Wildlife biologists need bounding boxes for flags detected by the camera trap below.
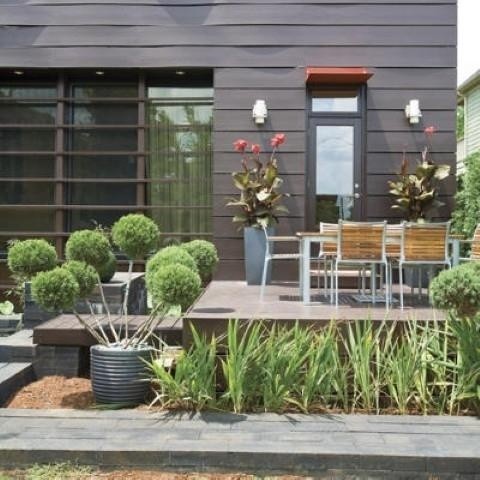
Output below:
[399,216,436,288]
[243,225,274,285]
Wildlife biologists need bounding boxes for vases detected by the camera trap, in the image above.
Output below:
[224,134,290,233]
[385,125,451,223]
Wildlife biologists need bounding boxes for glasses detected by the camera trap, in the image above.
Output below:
[404,99,422,124]
[253,98,268,124]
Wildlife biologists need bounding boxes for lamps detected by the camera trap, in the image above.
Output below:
[256,219,480,309]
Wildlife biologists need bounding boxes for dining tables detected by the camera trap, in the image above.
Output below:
[6,212,219,404]
